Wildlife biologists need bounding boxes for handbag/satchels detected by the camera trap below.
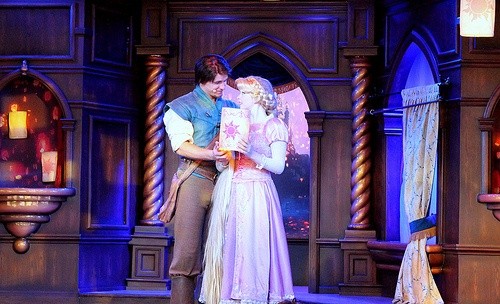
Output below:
[155,171,184,225]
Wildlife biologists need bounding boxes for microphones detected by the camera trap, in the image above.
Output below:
[204,85,217,93]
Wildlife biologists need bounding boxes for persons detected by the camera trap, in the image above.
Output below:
[199,76,296,304]
[158,55,240,303]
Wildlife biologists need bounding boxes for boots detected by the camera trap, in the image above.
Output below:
[168,274,194,303]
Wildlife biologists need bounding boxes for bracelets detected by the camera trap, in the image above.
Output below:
[255,153,267,170]
